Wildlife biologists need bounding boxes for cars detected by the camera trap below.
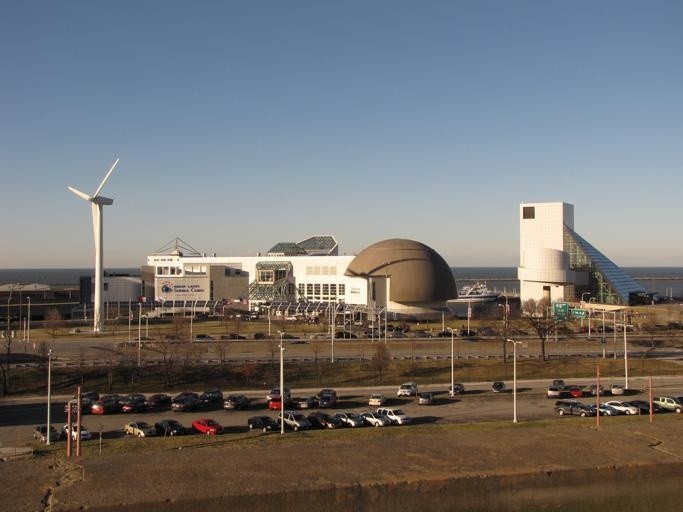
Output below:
[153,419,187,436]
[62,423,92,441]
[415,329,532,337]
[266,388,289,410]
[448,382,465,396]
[296,397,314,409]
[124,420,158,438]
[555,323,682,335]
[354,320,363,327]
[223,395,251,410]
[326,317,352,325]
[334,411,365,428]
[33,424,61,442]
[359,411,391,426]
[248,416,276,432]
[64,391,224,416]
[419,392,433,405]
[127,331,365,345]
[285,314,298,321]
[365,321,410,338]
[307,411,339,429]
[546,376,682,419]
[191,418,224,435]
[277,410,313,431]
[247,311,259,321]
[491,379,507,395]
[302,316,320,325]
[368,393,387,406]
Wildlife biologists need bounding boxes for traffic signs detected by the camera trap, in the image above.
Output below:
[552,302,590,321]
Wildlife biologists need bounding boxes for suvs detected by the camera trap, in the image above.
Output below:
[376,407,413,426]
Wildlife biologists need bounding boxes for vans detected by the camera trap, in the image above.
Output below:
[317,389,337,408]
[397,382,418,396]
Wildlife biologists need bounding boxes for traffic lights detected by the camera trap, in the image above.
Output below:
[24,295,33,344]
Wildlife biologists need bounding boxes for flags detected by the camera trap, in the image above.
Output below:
[0,330,8,339]
[158,296,165,304]
[139,296,149,303]
[9,330,17,339]
[222,298,232,305]
[240,297,248,305]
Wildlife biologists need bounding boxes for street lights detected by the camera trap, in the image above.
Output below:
[506,339,523,424]
[43,346,54,445]
[274,329,288,435]
[444,327,458,397]
[616,322,635,390]
[136,302,142,367]
[579,291,596,340]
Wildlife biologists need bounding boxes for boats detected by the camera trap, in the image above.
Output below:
[457,281,501,298]
